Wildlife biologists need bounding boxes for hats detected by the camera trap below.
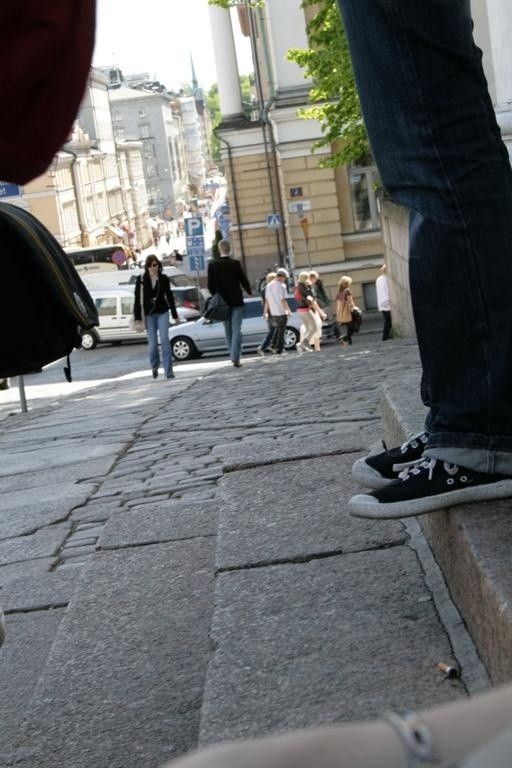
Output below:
[277,268,290,279]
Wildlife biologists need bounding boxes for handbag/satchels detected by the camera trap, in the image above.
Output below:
[336,299,352,324]
[129,314,146,333]
[202,292,230,320]
[0,201,100,377]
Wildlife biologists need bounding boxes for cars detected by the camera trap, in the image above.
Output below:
[197,187,217,214]
[148,292,341,361]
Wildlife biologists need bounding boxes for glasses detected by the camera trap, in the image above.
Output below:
[147,262,157,267]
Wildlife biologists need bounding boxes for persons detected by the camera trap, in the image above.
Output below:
[131,255,179,380]
[335,274,363,347]
[210,230,226,260]
[205,238,253,368]
[375,263,394,341]
[338,2,511,520]
[128,180,221,268]
[255,271,278,356]
[293,271,329,354]
[295,270,324,354]
[352,173,373,230]
[263,267,294,357]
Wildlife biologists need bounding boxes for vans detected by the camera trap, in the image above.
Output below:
[61,243,203,352]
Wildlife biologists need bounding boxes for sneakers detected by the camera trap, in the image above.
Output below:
[347,433,510,517]
[338,336,349,346]
[296,339,312,352]
[273,351,287,356]
[232,360,242,367]
[152,367,158,378]
[257,346,264,357]
[167,372,174,378]
